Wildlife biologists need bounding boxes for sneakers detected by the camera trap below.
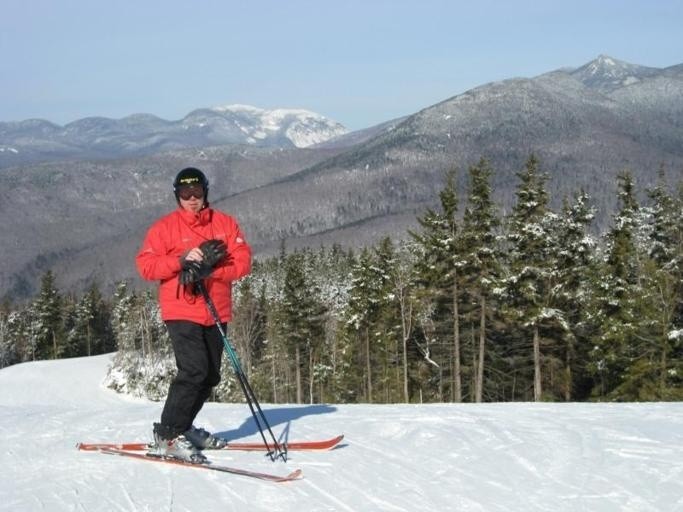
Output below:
[146,423,227,464]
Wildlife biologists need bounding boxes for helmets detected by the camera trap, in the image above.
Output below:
[173,168,208,192]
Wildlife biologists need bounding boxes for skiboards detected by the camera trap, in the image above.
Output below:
[77,431,345,481]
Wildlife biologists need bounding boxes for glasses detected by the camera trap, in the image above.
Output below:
[179,187,203,200]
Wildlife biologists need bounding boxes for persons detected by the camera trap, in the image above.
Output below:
[136,167,251,464]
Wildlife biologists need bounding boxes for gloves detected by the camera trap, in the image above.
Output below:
[179,239,226,286]
